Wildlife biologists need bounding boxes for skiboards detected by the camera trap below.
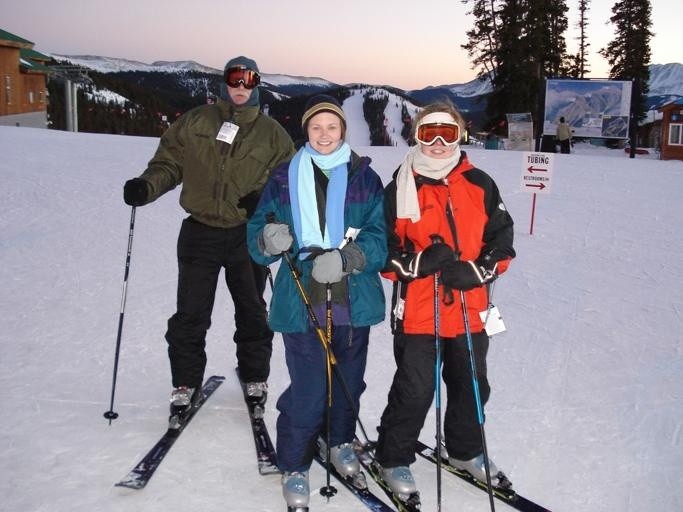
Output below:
[352,426,551,512]
[314,436,395,512]
[114,366,281,489]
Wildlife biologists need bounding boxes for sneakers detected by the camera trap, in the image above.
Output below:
[318,435,361,476]
[169,383,201,417]
[242,380,269,408]
[447,451,500,483]
[370,456,418,496]
[280,468,311,510]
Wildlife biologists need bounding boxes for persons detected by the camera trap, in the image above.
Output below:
[120,56,298,416]
[368,102,514,504]
[242,93,388,508]
[555,117,572,154]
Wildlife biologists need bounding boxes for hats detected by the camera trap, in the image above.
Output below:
[298,92,349,135]
[220,55,261,108]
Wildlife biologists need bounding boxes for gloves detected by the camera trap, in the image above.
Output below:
[439,251,500,292]
[122,176,150,208]
[235,188,264,221]
[390,241,457,286]
[257,221,296,258]
[310,240,368,286]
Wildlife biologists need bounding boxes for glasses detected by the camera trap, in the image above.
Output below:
[222,64,262,90]
[413,120,462,149]
